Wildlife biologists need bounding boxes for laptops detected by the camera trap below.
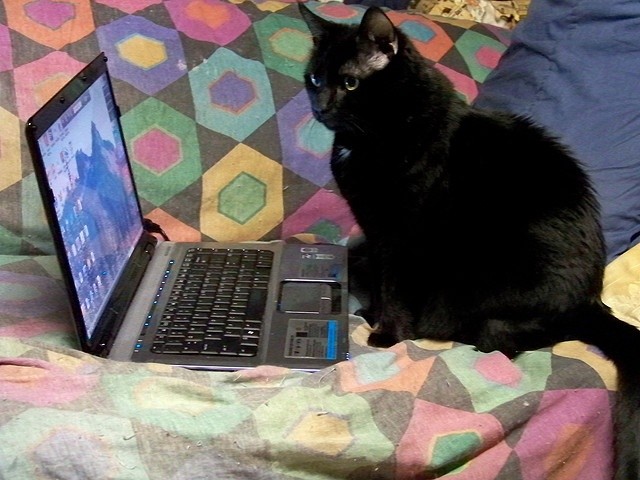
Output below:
[25,51,348,372]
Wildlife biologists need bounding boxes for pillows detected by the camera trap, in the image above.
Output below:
[469,1,640,263]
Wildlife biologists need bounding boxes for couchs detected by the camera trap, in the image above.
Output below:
[0,0,640,480]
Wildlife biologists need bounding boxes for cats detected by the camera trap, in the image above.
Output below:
[296,0,640,480]
